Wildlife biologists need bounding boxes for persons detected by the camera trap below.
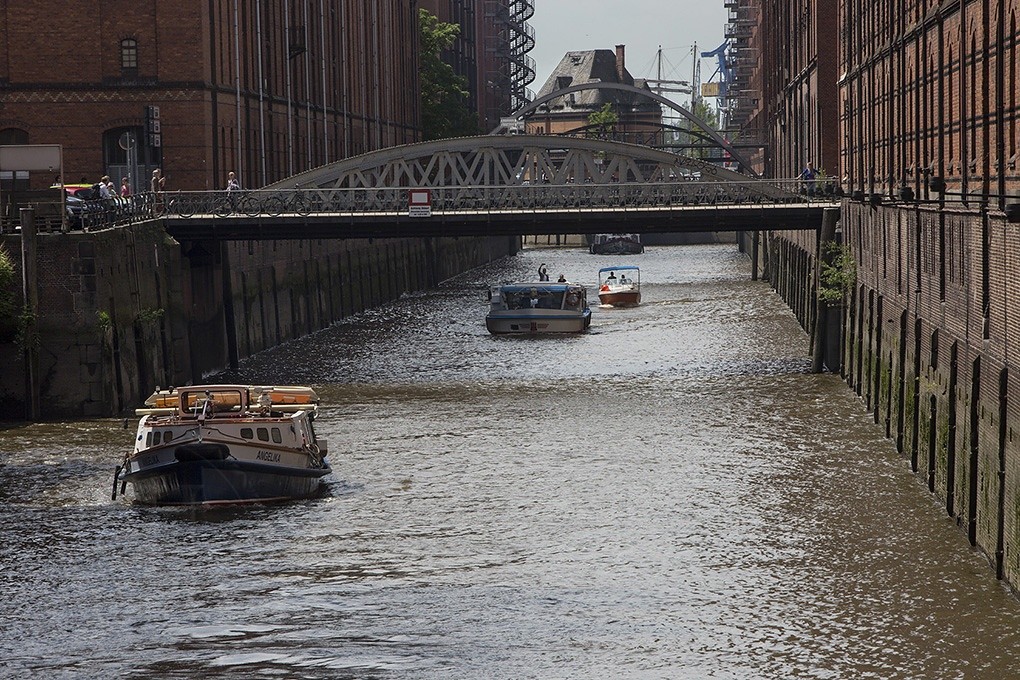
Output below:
[607,272,617,279]
[795,162,821,200]
[228,172,239,217]
[53,169,166,232]
[558,274,566,282]
[538,263,549,281]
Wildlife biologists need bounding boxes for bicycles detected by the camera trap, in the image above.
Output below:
[263,183,314,217]
[149,188,196,218]
[214,187,262,218]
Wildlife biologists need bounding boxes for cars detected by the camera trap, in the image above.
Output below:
[114,196,137,215]
[64,196,104,232]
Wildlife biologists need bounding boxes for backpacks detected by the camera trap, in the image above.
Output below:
[92,183,104,198]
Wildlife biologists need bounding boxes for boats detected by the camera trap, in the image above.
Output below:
[590,233,643,255]
[597,265,643,305]
[485,280,593,334]
[112,385,333,508]
